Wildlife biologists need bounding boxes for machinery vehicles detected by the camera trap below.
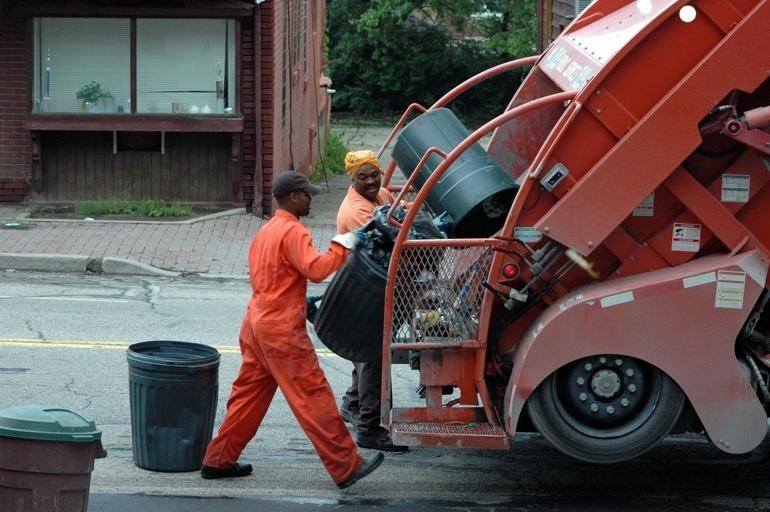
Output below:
[365,2,770,466]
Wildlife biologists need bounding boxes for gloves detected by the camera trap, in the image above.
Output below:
[306,295,322,323]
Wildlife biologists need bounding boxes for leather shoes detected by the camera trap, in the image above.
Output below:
[337,453,384,488]
[201,463,253,479]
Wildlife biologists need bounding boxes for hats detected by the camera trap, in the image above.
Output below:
[273,171,321,198]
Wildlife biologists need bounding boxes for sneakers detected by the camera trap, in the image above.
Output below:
[356,434,409,452]
[341,406,358,426]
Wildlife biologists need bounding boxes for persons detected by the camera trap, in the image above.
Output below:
[335,149,431,455]
[199,166,386,490]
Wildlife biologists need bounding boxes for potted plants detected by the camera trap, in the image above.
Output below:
[73,79,115,112]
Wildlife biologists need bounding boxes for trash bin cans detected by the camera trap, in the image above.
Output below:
[390,106,522,250]
[312,204,445,364]
[124,340,222,473]
[0,403,109,512]
